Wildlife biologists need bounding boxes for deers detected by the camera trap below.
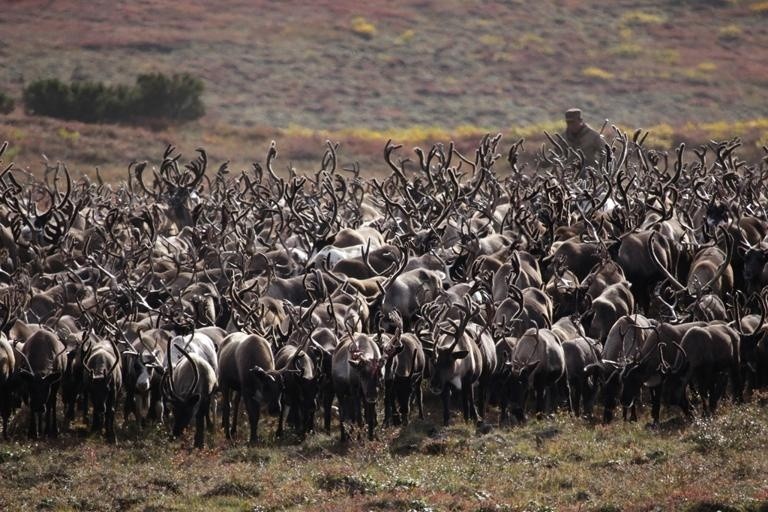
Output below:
[0,125,768,446]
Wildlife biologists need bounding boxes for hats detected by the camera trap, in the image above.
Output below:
[564,108,584,121]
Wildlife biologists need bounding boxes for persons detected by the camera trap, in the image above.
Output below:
[537,106,610,184]
